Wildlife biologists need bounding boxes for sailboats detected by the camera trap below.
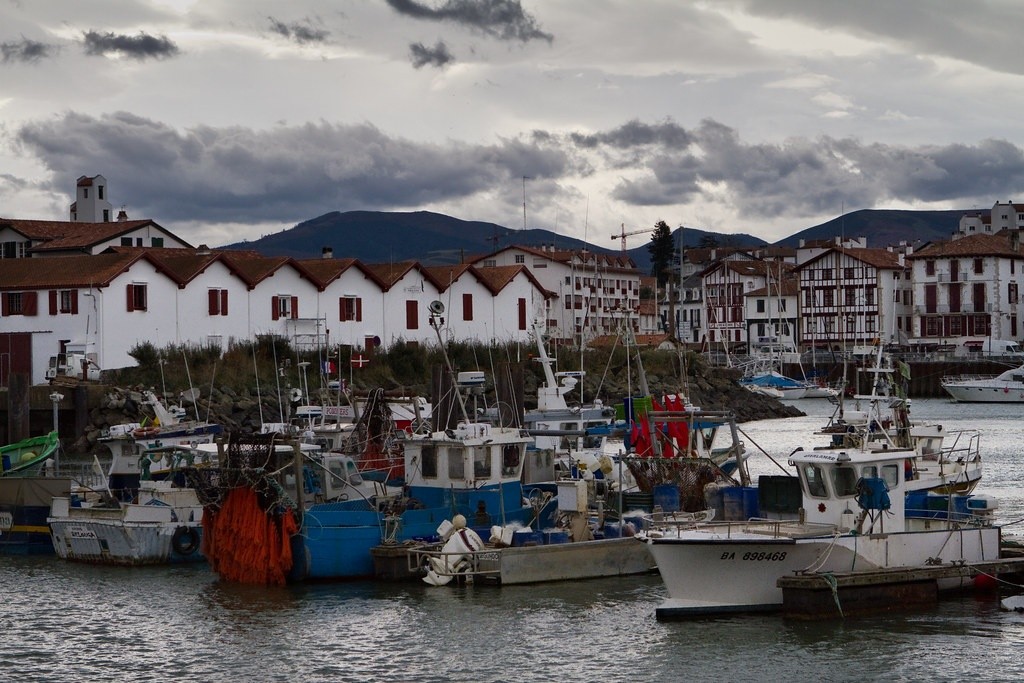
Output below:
[692,257,847,400]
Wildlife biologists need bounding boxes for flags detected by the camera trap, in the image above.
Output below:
[318,360,336,374]
[350,353,371,369]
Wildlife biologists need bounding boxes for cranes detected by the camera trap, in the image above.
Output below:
[611,223,657,253]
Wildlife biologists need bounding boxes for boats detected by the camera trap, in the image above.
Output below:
[643,271,1023,623]
[939,364,1023,402]
[0,194,766,585]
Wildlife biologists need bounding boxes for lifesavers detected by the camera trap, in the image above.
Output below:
[172,526,200,556]
[131,427,160,437]
